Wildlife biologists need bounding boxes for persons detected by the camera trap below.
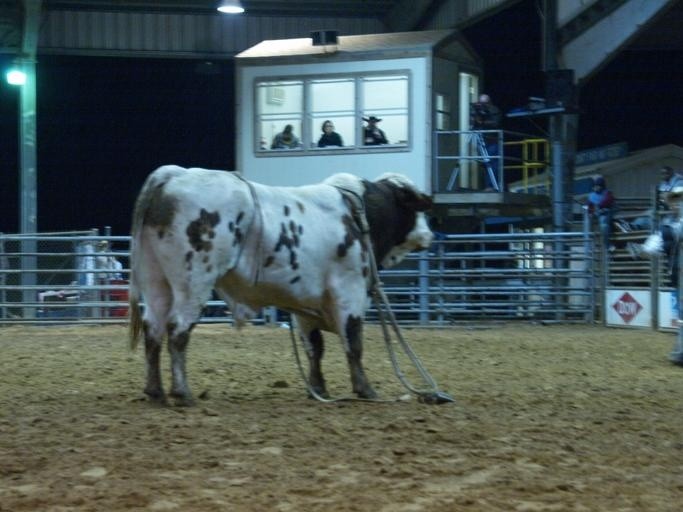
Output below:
[363,117,387,145]
[318,120,342,147]
[470,94,506,193]
[613,166,683,365]
[271,124,298,149]
[96,240,123,278]
[583,174,615,249]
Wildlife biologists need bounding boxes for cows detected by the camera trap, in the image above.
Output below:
[123,162,437,409]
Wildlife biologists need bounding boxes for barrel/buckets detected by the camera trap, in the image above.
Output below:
[109,279,130,316]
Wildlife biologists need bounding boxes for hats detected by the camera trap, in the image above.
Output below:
[361,116,382,123]
